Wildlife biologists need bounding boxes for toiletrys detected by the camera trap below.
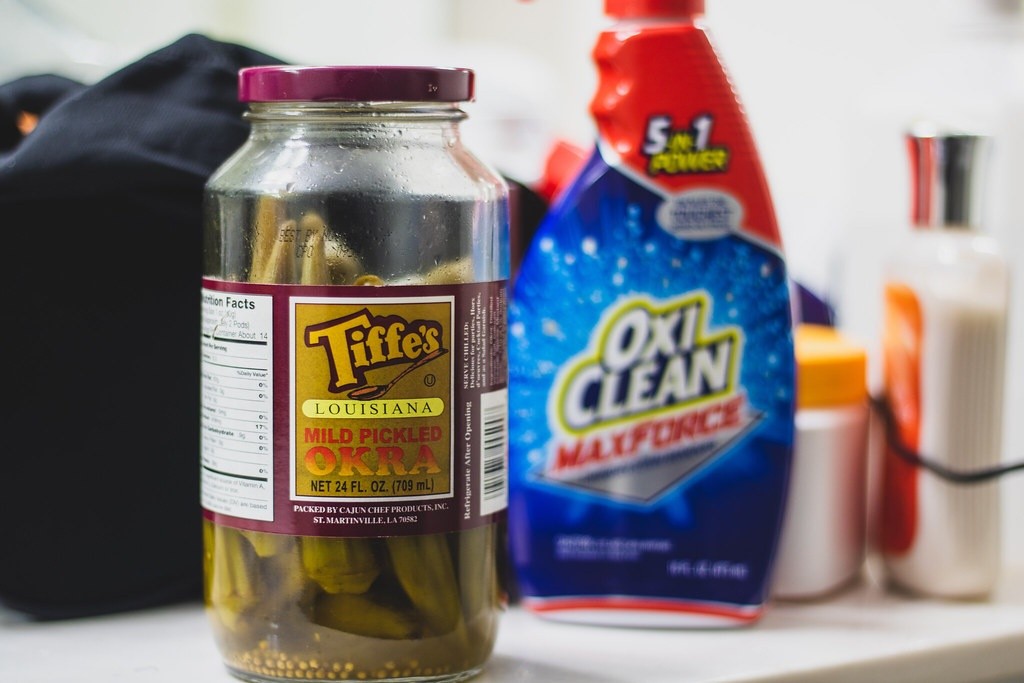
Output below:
[768,320,873,596]
[873,128,1011,601]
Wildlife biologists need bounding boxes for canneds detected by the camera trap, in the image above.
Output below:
[199,64,513,683]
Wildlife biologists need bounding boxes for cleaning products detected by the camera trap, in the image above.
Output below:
[504,0,797,628]
